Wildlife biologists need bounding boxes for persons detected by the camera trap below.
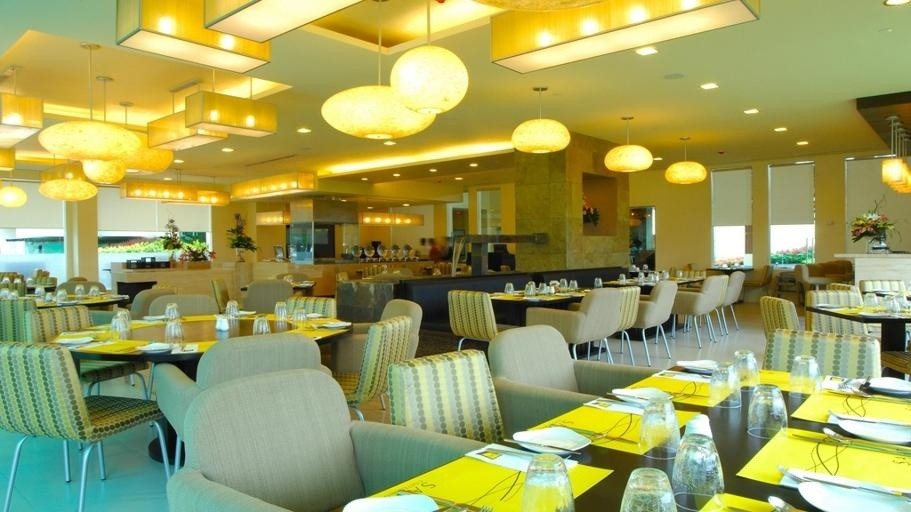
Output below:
[415,236,450,260]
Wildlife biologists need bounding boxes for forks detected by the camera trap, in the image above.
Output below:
[399,490,492,511]
[488,447,572,464]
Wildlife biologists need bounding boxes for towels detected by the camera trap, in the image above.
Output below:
[183,343,199,354]
[779,468,911,496]
[652,369,714,382]
[829,411,911,431]
[584,397,645,415]
[823,375,867,391]
[467,443,578,475]
[319,322,351,329]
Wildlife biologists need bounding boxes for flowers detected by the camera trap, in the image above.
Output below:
[223,213,266,253]
[582,191,602,227]
[844,209,904,242]
[159,217,182,249]
[180,245,217,263]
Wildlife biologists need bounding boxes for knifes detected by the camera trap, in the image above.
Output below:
[829,410,908,427]
[805,476,911,496]
[830,391,910,406]
[792,434,910,457]
[503,440,582,457]
[561,424,638,445]
[605,392,652,401]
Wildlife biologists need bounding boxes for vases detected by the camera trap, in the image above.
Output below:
[867,238,890,254]
[233,247,247,262]
[168,250,178,259]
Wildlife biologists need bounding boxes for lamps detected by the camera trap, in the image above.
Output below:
[121,167,198,202]
[604,117,654,172]
[184,66,276,138]
[470,1,602,12]
[203,0,376,45]
[82,77,124,184]
[145,81,229,151]
[888,123,907,185]
[37,43,142,160]
[115,1,270,75]
[889,128,909,188]
[161,176,229,207]
[2,67,43,149]
[893,134,910,190]
[665,137,707,184]
[256,201,291,226]
[490,3,760,74]
[109,102,173,177]
[359,207,424,226]
[39,153,98,202]
[228,160,315,201]
[881,115,902,183]
[512,86,571,153]
[390,1,467,113]
[321,25,436,141]
[896,138,911,193]
[3,148,29,208]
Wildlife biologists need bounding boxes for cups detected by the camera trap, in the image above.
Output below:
[648,272,654,282]
[252,319,271,338]
[560,278,567,289]
[165,320,184,342]
[11,293,18,304]
[638,272,644,282]
[539,283,547,294]
[90,286,100,299]
[672,434,725,512]
[884,292,910,317]
[164,303,182,321]
[733,350,758,390]
[570,281,578,290]
[274,302,288,323]
[111,315,132,341]
[639,398,681,461]
[117,308,132,331]
[56,288,68,304]
[790,355,824,401]
[524,284,533,297]
[594,278,603,289]
[529,282,536,295]
[224,301,240,320]
[747,384,789,440]
[34,286,44,296]
[292,308,307,332]
[75,285,85,297]
[676,271,682,278]
[709,360,741,410]
[521,452,575,512]
[620,467,675,511]
[504,283,514,294]
[619,274,627,285]
[654,273,659,281]
[662,272,669,280]
[864,293,879,311]
[2,288,8,299]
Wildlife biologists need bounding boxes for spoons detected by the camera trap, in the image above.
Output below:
[768,496,807,512]
[823,428,910,452]
[849,387,909,401]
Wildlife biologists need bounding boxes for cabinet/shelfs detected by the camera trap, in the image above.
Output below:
[223,251,467,296]
[106,260,236,310]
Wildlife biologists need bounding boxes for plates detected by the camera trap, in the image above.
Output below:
[813,303,844,310]
[523,296,544,303]
[341,494,438,512]
[610,387,674,405]
[838,418,911,445]
[324,321,352,329]
[141,314,165,322]
[58,337,93,346]
[859,312,891,318]
[110,294,127,299]
[858,376,910,396]
[136,342,172,354]
[238,310,257,317]
[58,300,80,306]
[556,293,574,297]
[306,312,321,318]
[798,480,911,512]
[676,359,719,373]
[513,425,592,456]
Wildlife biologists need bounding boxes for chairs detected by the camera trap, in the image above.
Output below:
[90,288,178,326]
[804,290,867,334]
[65,277,89,281]
[525,286,620,365]
[699,271,746,334]
[796,260,855,290]
[826,282,864,304]
[688,274,729,341]
[573,285,641,367]
[0,272,24,283]
[655,276,721,348]
[0,341,171,512]
[760,295,802,341]
[330,299,422,409]
[54,281,106,296]
[762,325,882,383]
[154,334,333,472]
[598,281,678,366]
[447,290,523,351]
[386,348,505,450]
[166,369,492,511]
[860,279,911,339]
[743,262,773,295]
[0,298,37,343]
[24,305,152,401]
[333,315,411,421]
[487,325,663,438]
